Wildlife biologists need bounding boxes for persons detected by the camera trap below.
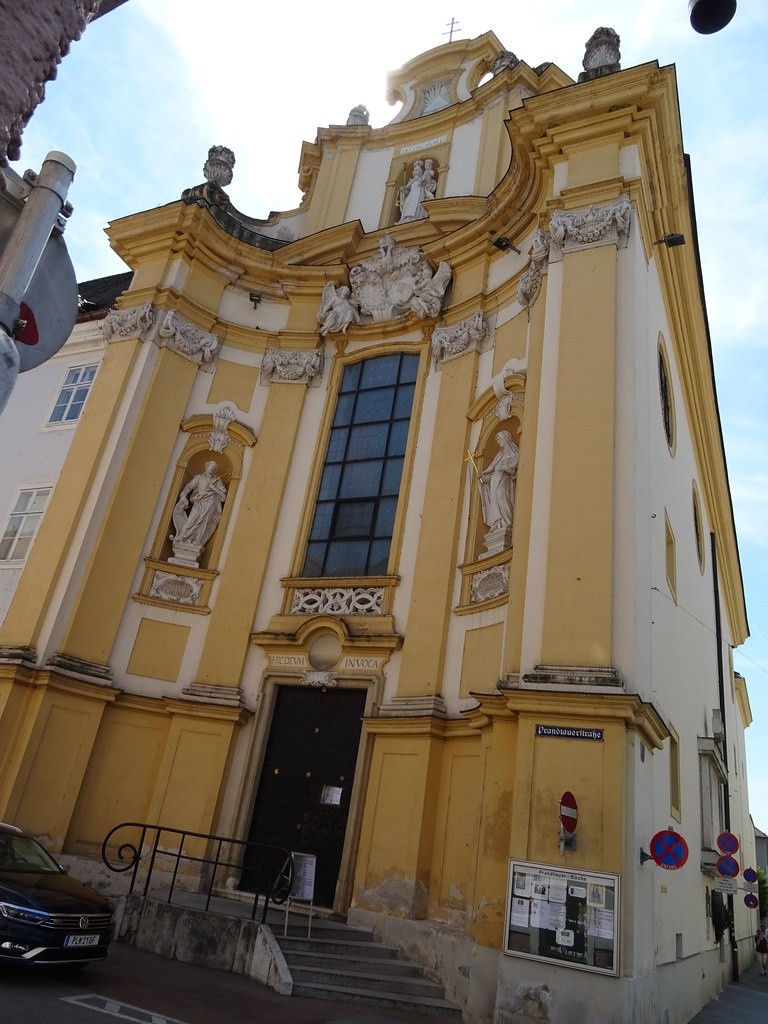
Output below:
[394,160,437,226]
[754,925,768,976]
[550,204,628,243]
[319,286,362,336]
[476,430,519,534]
[102,304,155,341]
[541,885,545,895]
[411,269,441,319]
[174,461,226,546]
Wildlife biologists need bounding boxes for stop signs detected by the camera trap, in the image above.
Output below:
[560,790,579,833]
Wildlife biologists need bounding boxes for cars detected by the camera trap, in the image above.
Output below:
[0,822,117,974]
